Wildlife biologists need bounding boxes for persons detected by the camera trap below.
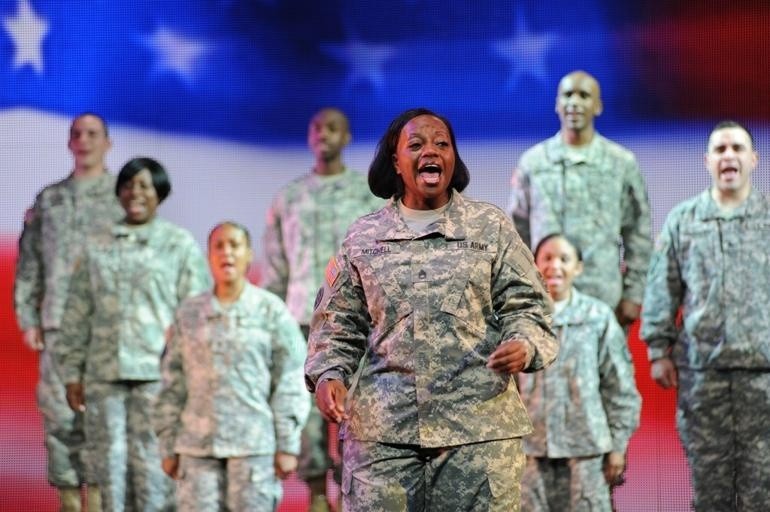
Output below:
[505,70,654,336]
[53,156,214,511]
[151,222,310,511]
[258,107,386,511]
[638,120,770,511]
[12,109,130,511]
[513,230,644,511]
[303,107,561,511]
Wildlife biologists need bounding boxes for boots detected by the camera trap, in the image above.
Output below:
[87,486,104,512]
[60,486,82,512]
[310,480,330,512]
